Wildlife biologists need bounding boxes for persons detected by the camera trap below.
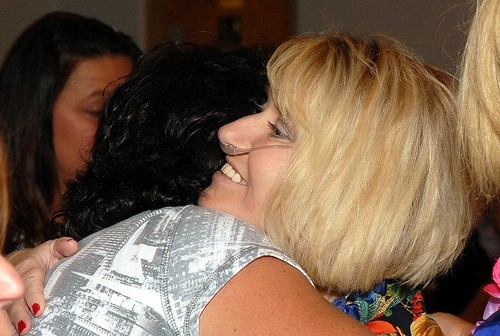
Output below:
[1,9,145,256]
[427,0,499,334]
[17,34,384,335]
[1,30,487,334]
[0,139,27,336]
